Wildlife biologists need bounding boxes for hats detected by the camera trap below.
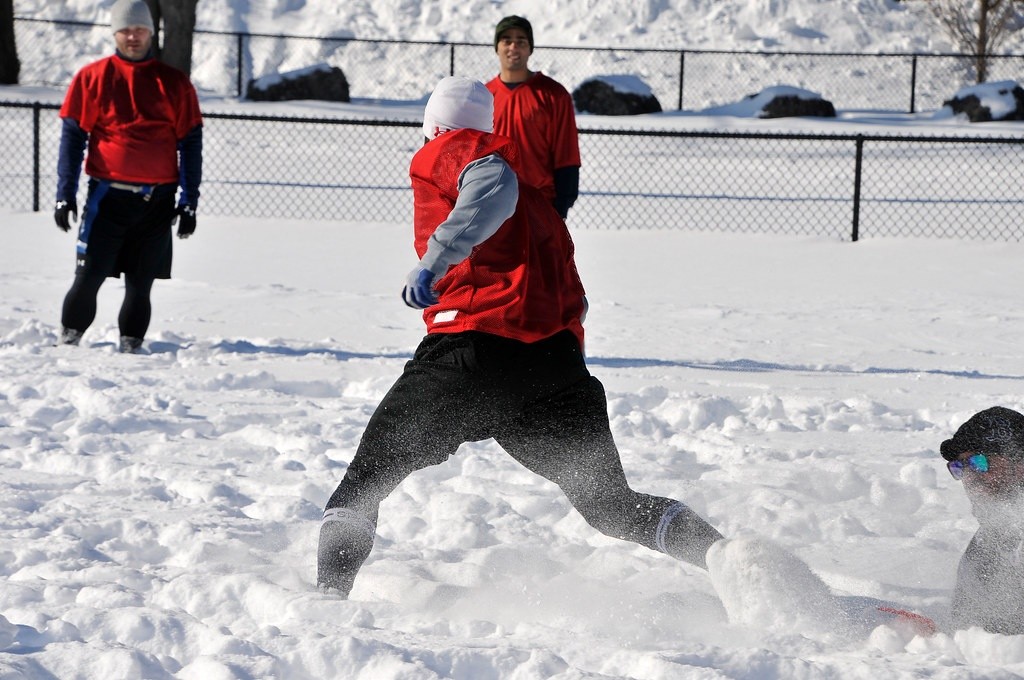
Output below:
[110,0,154,36]
[422,75,494,140]
[494,15,533,54]
[940,406,1024,462]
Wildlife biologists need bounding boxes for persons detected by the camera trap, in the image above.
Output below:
[316,75,726,599]
[54,0,202,353]
[839,406,1024,638]
[484,15,582,222]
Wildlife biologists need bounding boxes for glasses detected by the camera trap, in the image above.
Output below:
[946,452,989,481]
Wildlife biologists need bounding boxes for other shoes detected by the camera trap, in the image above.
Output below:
[53,328,83,347]
[118,335,145,355]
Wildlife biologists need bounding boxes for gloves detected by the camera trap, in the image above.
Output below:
[400,263,440,310]
[171,204,197,240]
[54,197,78,232]
